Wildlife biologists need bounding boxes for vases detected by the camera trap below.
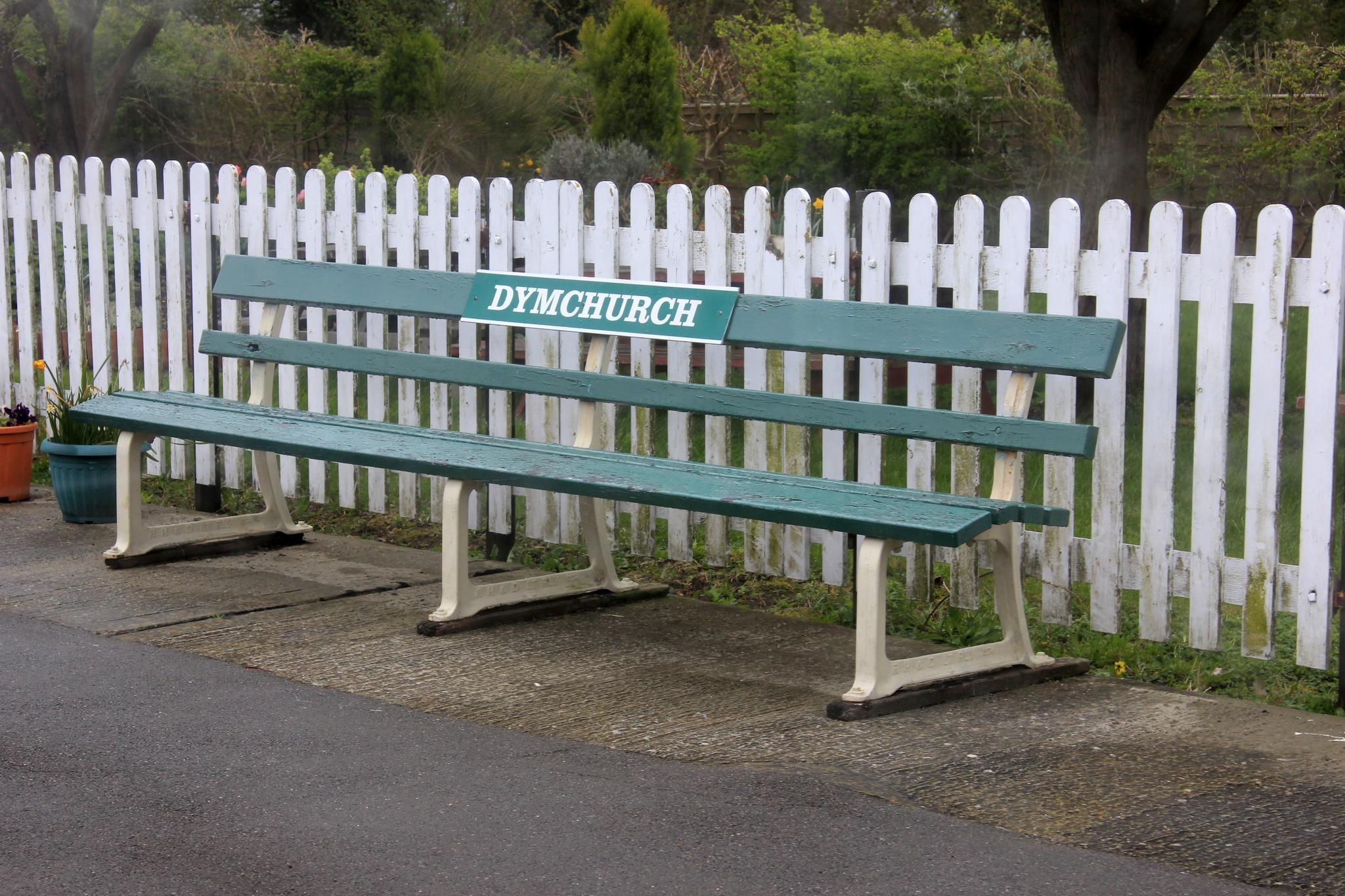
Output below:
[0,422,39,502]
[40,438,151,525]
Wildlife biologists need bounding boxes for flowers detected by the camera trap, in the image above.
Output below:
[0,402,38,428]
[34,355,162,463]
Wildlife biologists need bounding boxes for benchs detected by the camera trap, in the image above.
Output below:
[72,255,1127,718]
